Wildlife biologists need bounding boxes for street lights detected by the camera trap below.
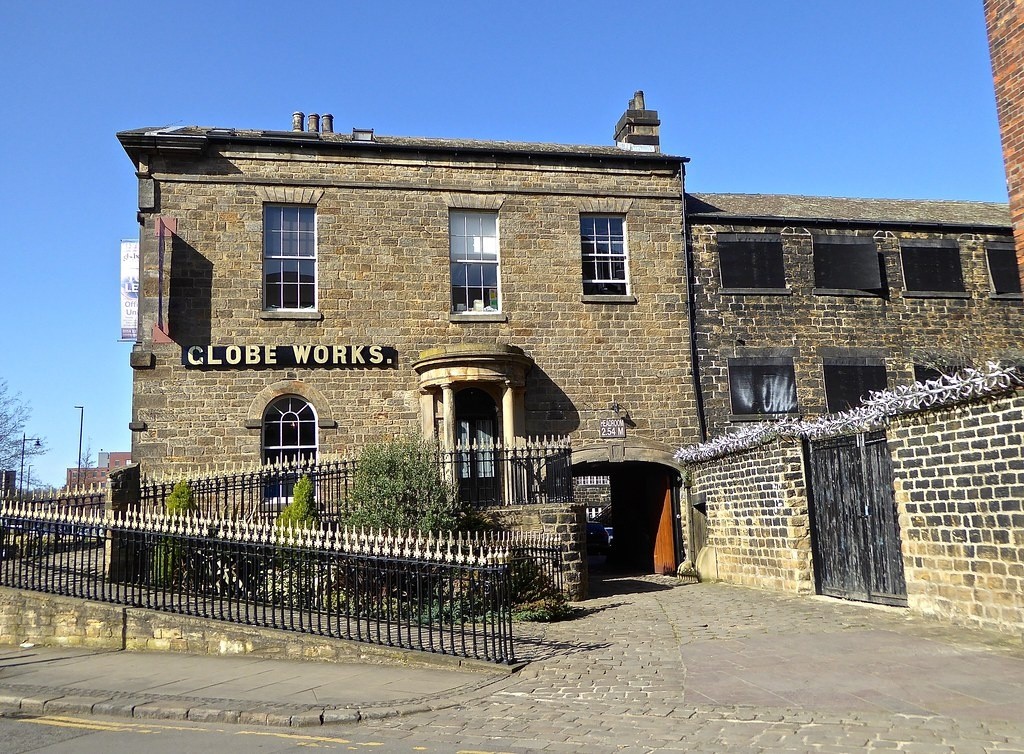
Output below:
[20,439,42,508]
[75,406,83,492]
[27,465,34,491]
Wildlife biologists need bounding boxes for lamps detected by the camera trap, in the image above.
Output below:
[610,399,636,429]
